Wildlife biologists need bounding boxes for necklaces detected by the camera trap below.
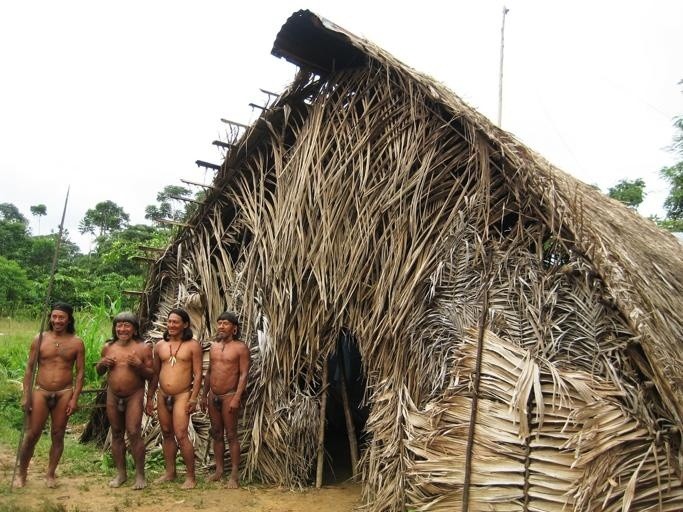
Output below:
[220,341,232,352]
[168,344,181,367]
[54,335,63,348]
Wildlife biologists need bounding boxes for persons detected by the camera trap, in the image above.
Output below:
[145,309,204,491]
[13,303,86,490]
[199,311,251,488]
[95,312,155,490]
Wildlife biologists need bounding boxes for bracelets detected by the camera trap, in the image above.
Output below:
[190,400,198,404]
